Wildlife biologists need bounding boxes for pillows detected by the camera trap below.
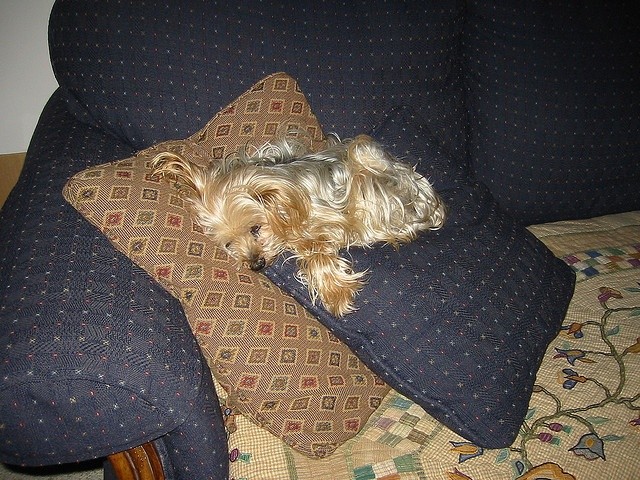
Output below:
[268,108,575,448]
[62,72,389,460]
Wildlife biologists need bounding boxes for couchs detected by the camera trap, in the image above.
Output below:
[0,0,639,480]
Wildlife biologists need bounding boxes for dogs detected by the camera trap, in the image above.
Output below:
[152,134,446,319]
